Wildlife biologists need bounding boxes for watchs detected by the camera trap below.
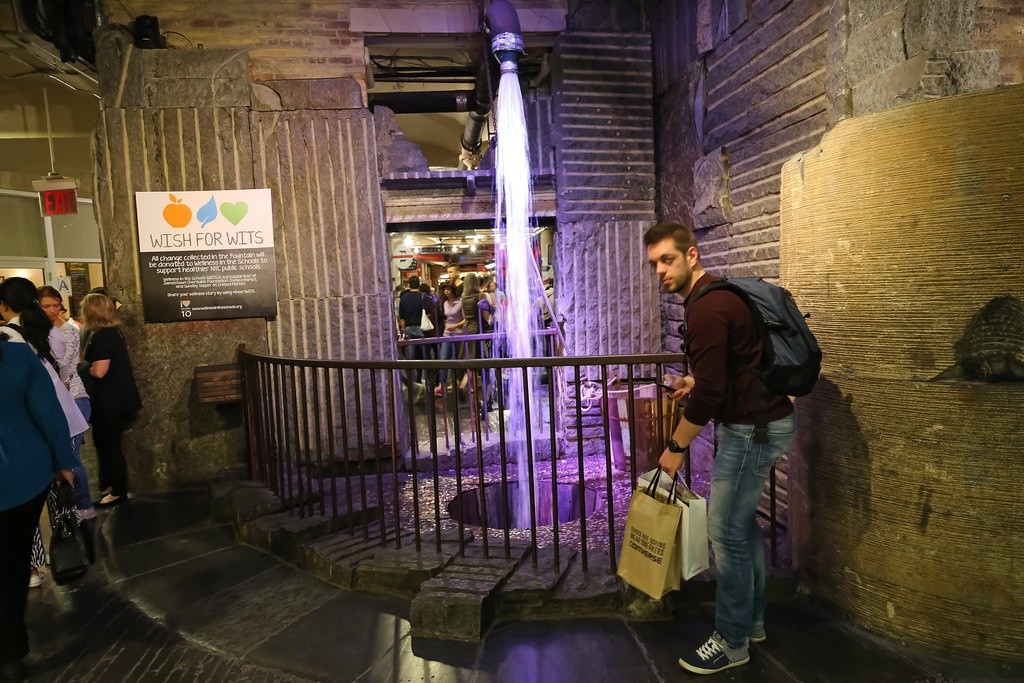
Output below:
[669,440,688,453]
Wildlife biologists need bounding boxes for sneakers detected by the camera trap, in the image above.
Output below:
[678,630,750,675]
[746,635,766,643]
[435,387,442,395]
[412,383,424,403]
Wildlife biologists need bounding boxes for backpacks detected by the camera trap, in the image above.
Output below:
[681,275,824,398]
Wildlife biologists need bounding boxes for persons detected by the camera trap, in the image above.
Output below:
[77,293,143,509]
[393,263,554,406]
[0,276,105,588]
[0,332,77,683]
[643,223,797,674]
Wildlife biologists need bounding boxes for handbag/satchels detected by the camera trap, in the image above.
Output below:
[420,309,434,330]
[616,463,710,600]
[49,480,88,587]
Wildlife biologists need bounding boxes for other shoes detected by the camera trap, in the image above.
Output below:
[0,662,26,683]
[459,386,463,392]
[93,494,128,508]
[447,386,452,389]
[28,576,42,587]
[76,507,95,519]
